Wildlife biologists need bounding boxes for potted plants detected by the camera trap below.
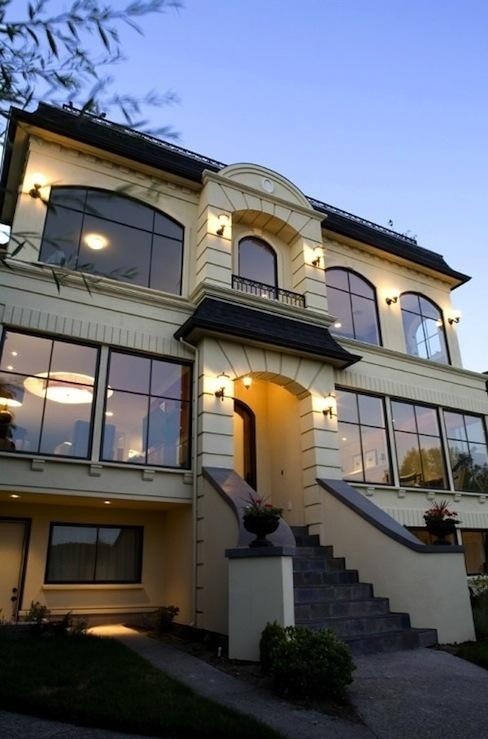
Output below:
[13,426,26,451]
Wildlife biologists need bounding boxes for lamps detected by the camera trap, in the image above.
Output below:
[29,172,47,199]
[213,365,254,401]
[447,309,462,324]
[312,244,326,269]
[319,390,337,420]
[215,212,232,237]
[40,375,94,405]
[0,383,26,425]
[386,288,400,306]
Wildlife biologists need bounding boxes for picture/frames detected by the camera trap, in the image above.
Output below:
[351,448,377,471]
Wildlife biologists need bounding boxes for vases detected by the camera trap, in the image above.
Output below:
[243,517,279,549]
[425,525,456,545]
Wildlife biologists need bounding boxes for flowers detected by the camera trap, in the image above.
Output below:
[422,497,465,525]
[239,491,285,517]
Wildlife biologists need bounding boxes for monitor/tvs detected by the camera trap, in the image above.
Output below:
[142,406,169,452]
